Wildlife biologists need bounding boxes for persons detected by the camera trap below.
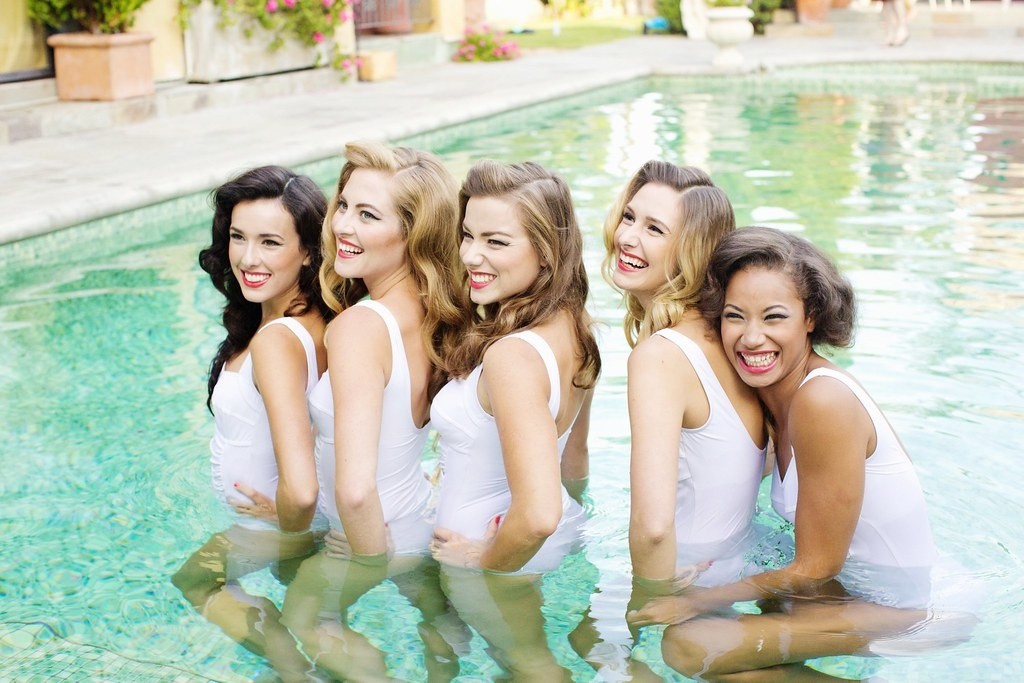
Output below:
[872,0,910,47]
[172,137,982,683]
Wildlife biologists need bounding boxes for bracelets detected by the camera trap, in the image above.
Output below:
[277,524,313,537]
[353,547,389,557]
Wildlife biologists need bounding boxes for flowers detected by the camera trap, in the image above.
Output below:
[173,0,364,84]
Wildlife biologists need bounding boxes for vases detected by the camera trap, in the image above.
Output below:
[184,0,357,84]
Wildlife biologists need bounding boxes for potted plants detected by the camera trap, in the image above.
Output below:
[704,0,755,67]
[27,0,157,104]
